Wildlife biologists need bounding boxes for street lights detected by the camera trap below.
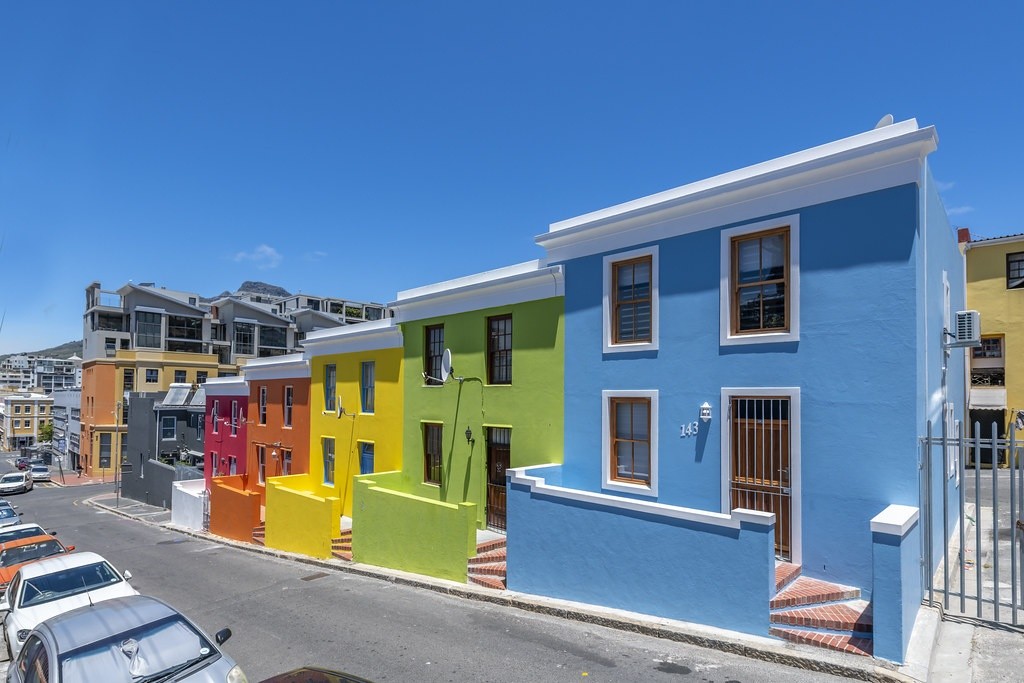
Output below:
[111,401,122,509]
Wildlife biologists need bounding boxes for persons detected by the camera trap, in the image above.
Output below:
[77,464,83,478]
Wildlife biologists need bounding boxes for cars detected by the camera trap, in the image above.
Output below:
[0,471,33,496]
[14,457,44,470]
[6,576,248,683]
[30,466,51,481]
[0,497,24,528]
[0,552,140,664]
[0,522,75,594]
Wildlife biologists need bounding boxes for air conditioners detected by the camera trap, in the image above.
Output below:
[955,310,982,342]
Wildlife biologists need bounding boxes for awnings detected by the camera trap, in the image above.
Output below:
[968,389,1007,410]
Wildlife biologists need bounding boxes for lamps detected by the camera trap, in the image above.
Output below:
[271,450,279,462]
[465,426,475,444]
[700,401,712,422]
[221,457,227,465]
